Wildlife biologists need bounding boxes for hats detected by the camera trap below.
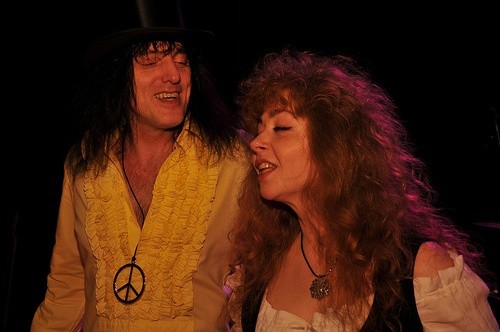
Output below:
[83,0,214,74]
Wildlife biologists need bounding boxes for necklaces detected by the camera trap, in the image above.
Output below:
[300,232,339,298]
[112,122,147,305]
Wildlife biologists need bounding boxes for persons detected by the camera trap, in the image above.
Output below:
[217,50,500,332]
[29,26,252,332]
